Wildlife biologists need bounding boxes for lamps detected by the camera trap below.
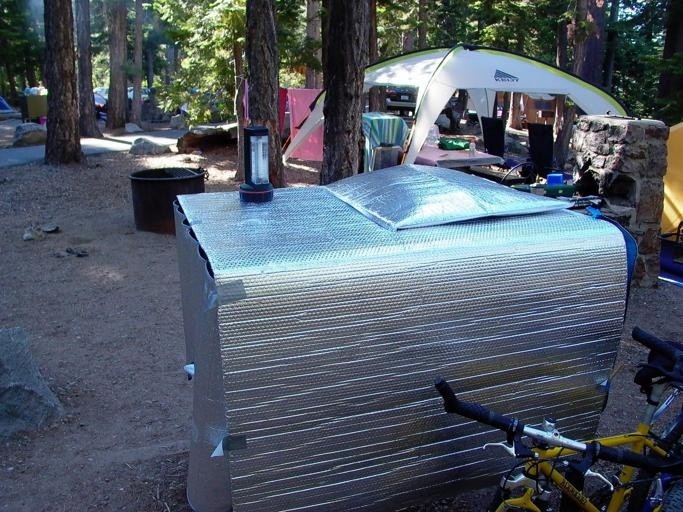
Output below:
[239,118,273,202]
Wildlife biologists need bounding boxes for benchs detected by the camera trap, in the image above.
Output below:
[467,116,505,170]
[498,123,554,185]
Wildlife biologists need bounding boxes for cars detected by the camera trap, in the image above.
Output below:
[385,88,416,115]
[90,86,152,112]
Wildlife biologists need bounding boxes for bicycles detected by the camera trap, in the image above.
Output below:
[436,328,683,512]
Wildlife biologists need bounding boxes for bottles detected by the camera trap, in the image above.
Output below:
[468,139,477,157]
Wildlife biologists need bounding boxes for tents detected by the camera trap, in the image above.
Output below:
[653,123,683,286]
[0,95,14,112]
[283,41,627,165]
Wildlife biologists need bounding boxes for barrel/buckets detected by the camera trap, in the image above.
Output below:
[127,166,207,234]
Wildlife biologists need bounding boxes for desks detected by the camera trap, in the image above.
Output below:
[418,148,503,167]
